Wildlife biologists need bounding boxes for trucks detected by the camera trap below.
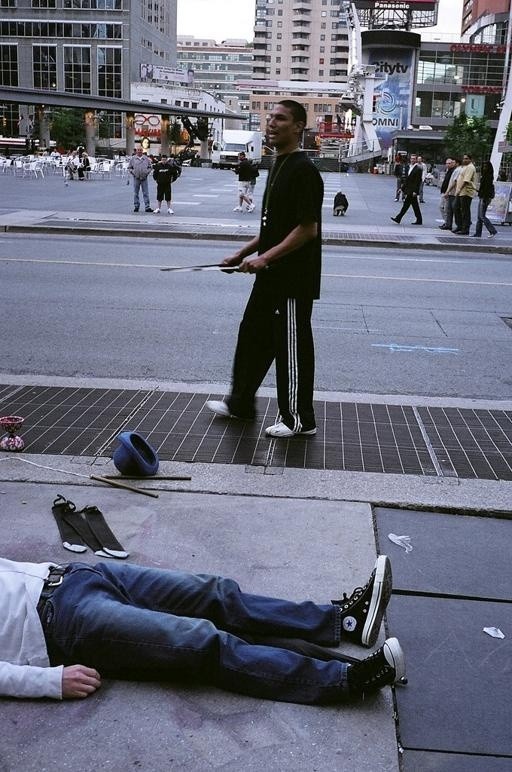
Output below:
[211,130,262,172]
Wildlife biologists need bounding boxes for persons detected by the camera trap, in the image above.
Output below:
[390,152,508,238]
[248,158,260,203]
[131,147,182,178]
[44,144,91,181]
[142,64,153,78]
[336,114,342,132]
[186,69,194,84]
[152,155,177,215]
[204,99,324,438]
[334,191,349,216]
[233,152,255,212]
[0,551,406,704]
[129,147,154,212]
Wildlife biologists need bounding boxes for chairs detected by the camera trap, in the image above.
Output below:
[0,152,130,179]
[180,158,192,175]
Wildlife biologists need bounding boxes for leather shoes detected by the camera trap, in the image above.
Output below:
[451,228,469,235]
[391,216,400,224]
[411,222,423,225]
[439,224,452,230]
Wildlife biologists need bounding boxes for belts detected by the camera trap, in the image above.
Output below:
[33,564,69,615]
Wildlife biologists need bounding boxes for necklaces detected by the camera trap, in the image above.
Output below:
[262,156,290,226]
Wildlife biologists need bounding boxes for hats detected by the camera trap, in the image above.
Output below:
[112,428,159,475]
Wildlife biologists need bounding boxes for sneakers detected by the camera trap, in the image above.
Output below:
[264,419,317,438]
[145,208,154,212]
[205,399,257,420]
[470,232,495,238]
[153,208,161,214]
[233,206,243,212]
[168,208,174,214]
[331,553,392,649]
[133,207,139,212]
[246,202,256,212]
[348,636,407,709]
